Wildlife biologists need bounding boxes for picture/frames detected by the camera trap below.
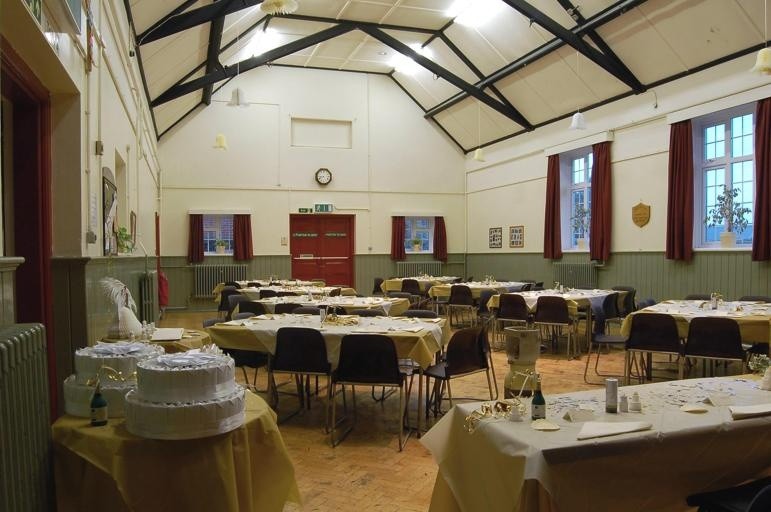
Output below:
[63,0,82,34]
[102,175,118,256]
[130,211,136,243]
[509,226,524,248]
[489,228,502,248]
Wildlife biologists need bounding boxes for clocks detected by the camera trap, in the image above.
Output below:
[315,168,332,185]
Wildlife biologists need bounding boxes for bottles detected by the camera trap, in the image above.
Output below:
[711,293,717,309]
[531,378,547,420]
[619,395,629,411]
[606,377,618,413]
[90,384,107,426]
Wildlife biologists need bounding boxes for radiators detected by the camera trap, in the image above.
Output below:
[0,322,57,511]
[140,269,160,328]
[194,264,248,299]
[396,261,442,278]
[552,262,598,291]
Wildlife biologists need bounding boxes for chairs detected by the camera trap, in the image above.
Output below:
[401,279,421,309]
[389,290,412,303]
[268,282,282,286]
[533,282,545,291]
[224,281,242,289]
[259,290,277,300]
[399,309,438,319]
[402,315,499,433]
[603,292,621,336]
[520,284,532,292]
[612,285,636,318]
[350,309,386,318]
[493,294,534,352]
[238,301,266,315]
[293,306,321,315]
[476,289,498,325]
[446,285,473,328]
[331,334,412,452]
[277,291,297,298]
[372,278,385,297]
[450,278,463,284]
[217,289,240,319]
[681,317,747,379]
[464,276,473,283]
[624,313,681,380]
[584,304,646,386]
[534,296,581,361]
[218,348,279,413]
[388,276,403,280]
[247,283,261,288]
[267,327,348,434]
[274,303,303,314]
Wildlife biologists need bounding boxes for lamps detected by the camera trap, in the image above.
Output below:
[472,100,486,162]
[568,51,589,131]
[225,28,250,109]
[212,87,227,151]
[749,0,771,75]
[260,0,298,16]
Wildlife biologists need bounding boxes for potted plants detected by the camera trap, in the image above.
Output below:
[702,184,752,249]
[214,240,226,254]
[569,209,591,250]
[413,238,421,251]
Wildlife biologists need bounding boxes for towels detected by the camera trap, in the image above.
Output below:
[351,323,387,333]
[577,420,652,439]
[728,404,771,420]
